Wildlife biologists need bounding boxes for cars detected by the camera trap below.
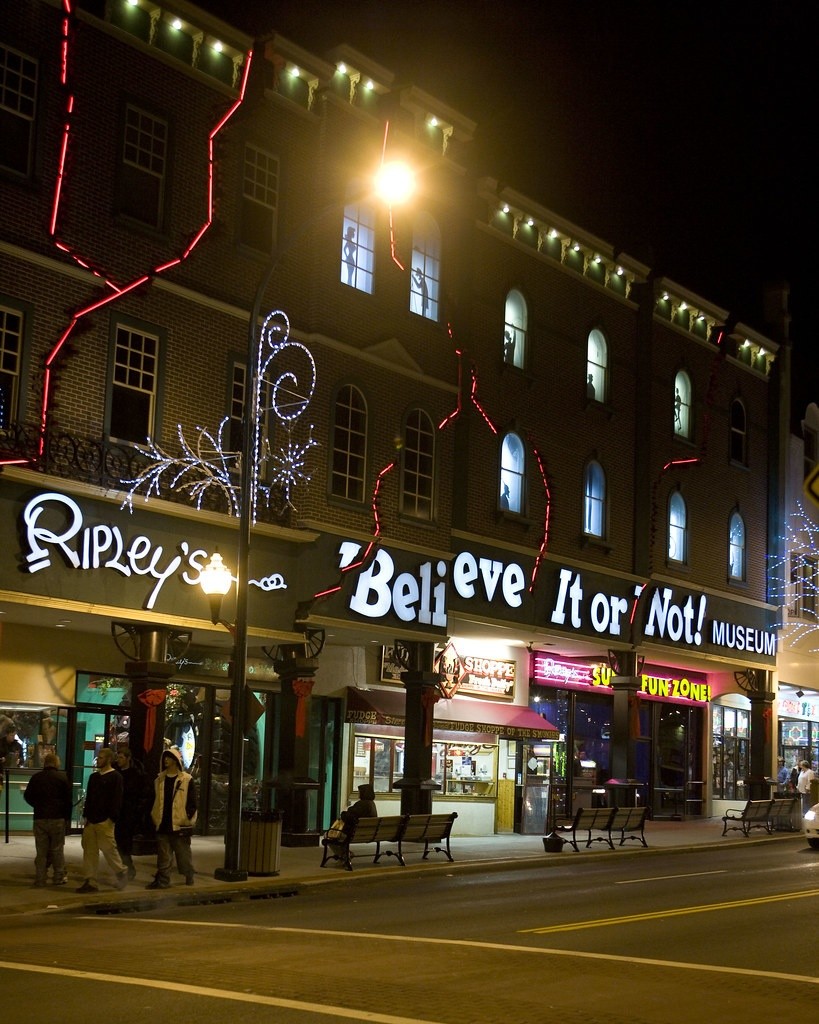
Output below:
[804,803,819,849]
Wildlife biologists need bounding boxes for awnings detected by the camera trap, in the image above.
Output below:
[346,685,559,741]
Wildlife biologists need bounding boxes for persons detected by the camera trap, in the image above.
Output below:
[327,784,377,867]
[77,748,127,891]
[111,750,148,879]
[778,756,815,818]
[146,749,196,889]
[0,726,24,767]
[24,753,73,888]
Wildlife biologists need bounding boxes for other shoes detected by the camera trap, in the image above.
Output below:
[128,867,136,880]
[75,882,99,893]
[144,878,168,890]
[52,876,68,886]
[114,873,130,890]
[185,875,194,886]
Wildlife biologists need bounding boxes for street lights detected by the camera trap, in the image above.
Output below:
[198,159,419,883]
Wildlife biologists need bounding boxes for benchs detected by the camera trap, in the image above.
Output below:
[552,805,653,853]
[320,812,459,871]
[722,796,799,838]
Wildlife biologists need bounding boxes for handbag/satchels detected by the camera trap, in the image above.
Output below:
[327,818,348,843]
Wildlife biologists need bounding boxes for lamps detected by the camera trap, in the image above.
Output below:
[796,690,804,697]
